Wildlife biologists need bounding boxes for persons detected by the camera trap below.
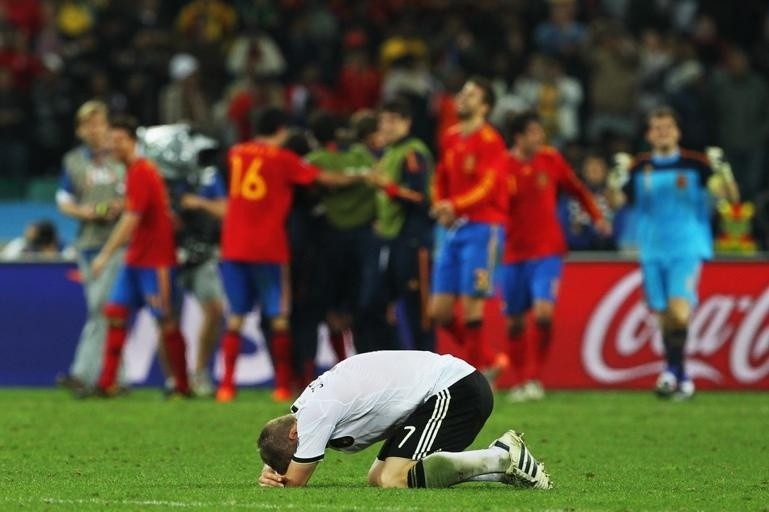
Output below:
[255,349,554,491]
[0,1,769,400]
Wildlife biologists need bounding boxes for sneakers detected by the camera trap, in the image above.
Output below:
[489,429,549,488]
